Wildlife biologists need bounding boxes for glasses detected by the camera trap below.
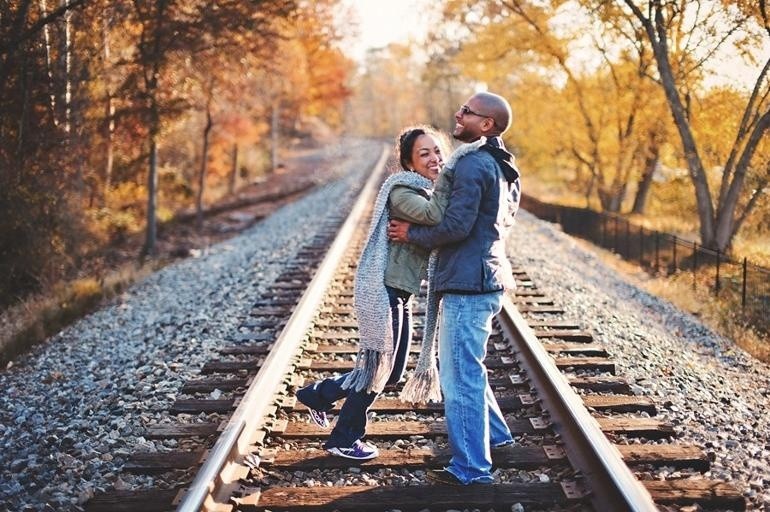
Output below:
[461,103,490,120]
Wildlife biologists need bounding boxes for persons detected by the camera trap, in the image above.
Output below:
[295,124,486,460]
[387,91,522,486]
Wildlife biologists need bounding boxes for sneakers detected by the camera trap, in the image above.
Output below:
[325,438,380,460]
[296,387,331,428]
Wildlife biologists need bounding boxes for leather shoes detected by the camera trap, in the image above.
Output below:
[491,438,516,453]
[425,466,490,486]
